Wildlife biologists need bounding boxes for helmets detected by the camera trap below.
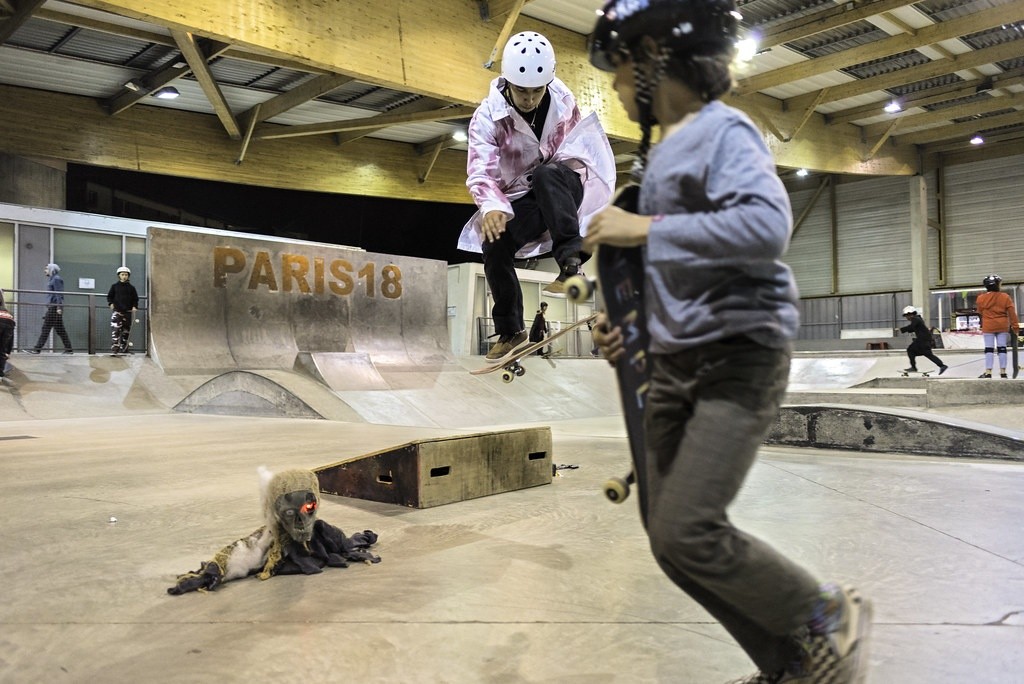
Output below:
[504,30,557,88]
[984,275,1003,286]
[902,305,918,316]
[590,0,739,75]
[116,266,131,276]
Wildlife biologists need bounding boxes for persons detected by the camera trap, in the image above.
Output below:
[587,320,599,358]
[894,306,948,375]
[21,263,73,355]
[529,302,549,356]
[457,31,616,363]
[975,274,1019,379]
[0,289,16,377]
[107,267,140,353]
[578,0,872,684]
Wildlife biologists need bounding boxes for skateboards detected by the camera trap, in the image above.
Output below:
[897,369,935,378]
[1010,324,1024,379]
[540,348,564,360]
[564,184,652,535]
[122,310,139,353]
[467,313,597,383]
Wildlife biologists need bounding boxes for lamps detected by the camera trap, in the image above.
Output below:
[123,77,150,96]
[151,86,180,100]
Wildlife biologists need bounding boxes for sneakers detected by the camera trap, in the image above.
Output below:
[805,587,874,684]
[725,659,810,684]
[486,329,528,363]
[555,262,584,286]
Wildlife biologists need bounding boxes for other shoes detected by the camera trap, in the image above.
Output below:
[23,349,40,356]
[111,344,119,353]
[590,351,598,358]
[904,367,917,372]
[979,372,992,378]
[939,365,948,375]
[1001,374,1007,378]
[61,350,73,355]
[119,348,129,353]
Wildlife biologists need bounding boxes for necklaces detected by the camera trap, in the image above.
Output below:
[506,89,536,128]
